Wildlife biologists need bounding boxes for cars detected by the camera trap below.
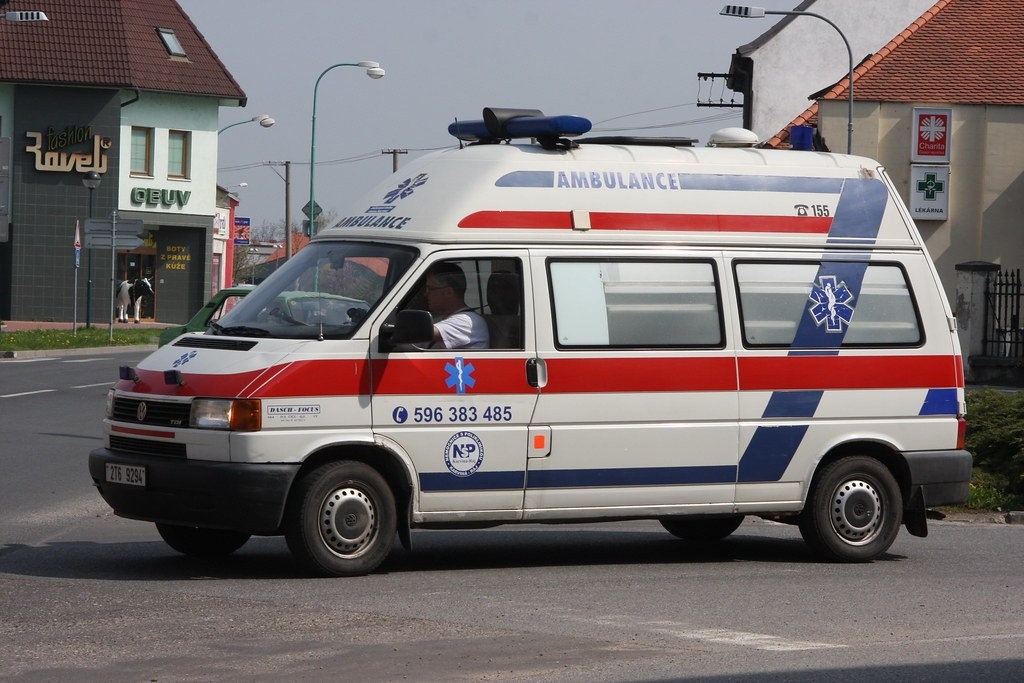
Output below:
[157,285,377,351]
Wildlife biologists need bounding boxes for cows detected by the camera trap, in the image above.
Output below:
[114,276,155,324]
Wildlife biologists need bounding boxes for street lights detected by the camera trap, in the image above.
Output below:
[309,61,387,307]
[719,2,852,153]
[83,171,101,330]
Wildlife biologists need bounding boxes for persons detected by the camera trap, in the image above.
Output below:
[410,263,490,350]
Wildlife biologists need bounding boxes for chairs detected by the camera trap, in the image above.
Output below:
[485,272,522,349]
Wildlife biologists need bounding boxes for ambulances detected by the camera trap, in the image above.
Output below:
[86,105,977,577]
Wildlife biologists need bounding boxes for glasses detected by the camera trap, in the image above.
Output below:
[420,285,451,294]
